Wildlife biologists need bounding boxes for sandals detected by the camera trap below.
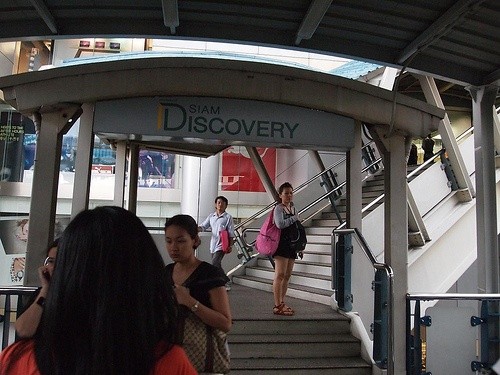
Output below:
[281,302,295,313]
[273,305,293,316]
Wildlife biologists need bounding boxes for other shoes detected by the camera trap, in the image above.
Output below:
[225,285,231,291]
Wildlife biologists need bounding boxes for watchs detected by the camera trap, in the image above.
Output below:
[190,301,200,313]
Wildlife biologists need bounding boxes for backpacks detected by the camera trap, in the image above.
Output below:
[282,204,307,253]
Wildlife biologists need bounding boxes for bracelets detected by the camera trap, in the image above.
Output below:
[35,296,47,308]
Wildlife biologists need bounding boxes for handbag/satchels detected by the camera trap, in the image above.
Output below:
[177,315,230,375]
[220,230,232,253]
[255,205,281,257]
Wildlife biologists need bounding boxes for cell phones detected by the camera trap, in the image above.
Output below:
[46,271,50,281]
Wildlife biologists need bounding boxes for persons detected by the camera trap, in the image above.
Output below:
[14,238,59,339]
[421,134,435,163]
[272,182,304,316]
[404,136,417,166]
[0,206,201,375]
[162,214,232,375]
[198,196,235,291]
